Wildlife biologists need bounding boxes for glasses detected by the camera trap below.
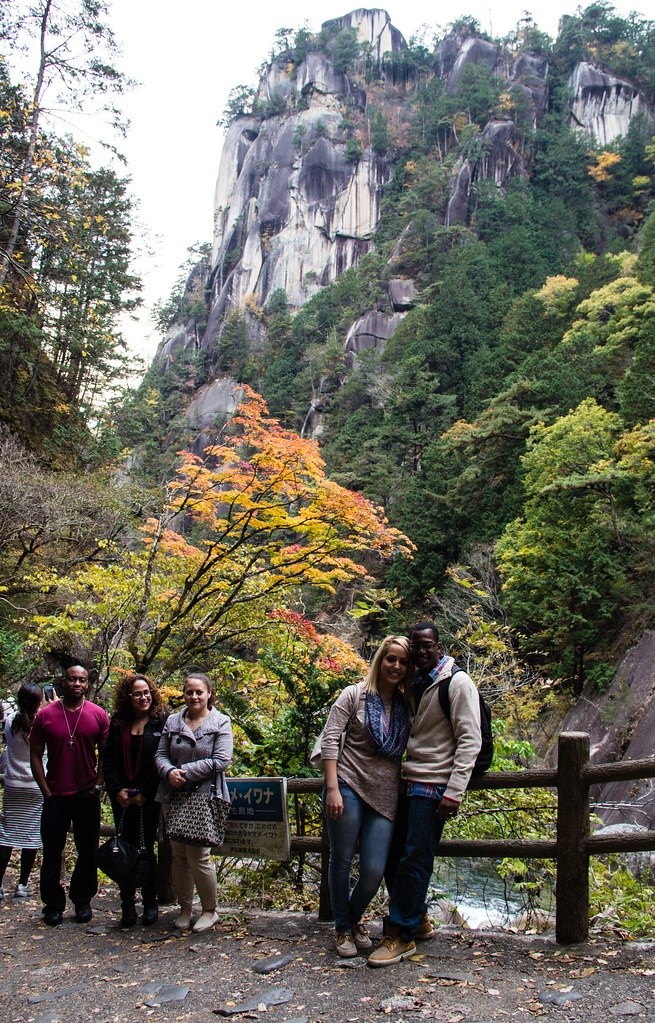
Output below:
[127,690,153,699]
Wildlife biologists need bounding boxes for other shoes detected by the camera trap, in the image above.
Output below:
[193,910,220,932]
[174,909,191,929]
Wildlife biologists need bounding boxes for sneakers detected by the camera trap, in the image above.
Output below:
[335,932,358,957]
[0,889,4,898]
[352,924,373,950]
[44,909,62,925]
[15,882,33,896]
[366,934,418,966]
[383,908,430,940]
[76,905,92,923]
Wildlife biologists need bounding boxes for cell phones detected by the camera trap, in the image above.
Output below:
[44,684,53,702]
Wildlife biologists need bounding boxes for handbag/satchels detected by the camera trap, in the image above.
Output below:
[309,683,361,771]
[96,803,155,888]
[166,758,230,848]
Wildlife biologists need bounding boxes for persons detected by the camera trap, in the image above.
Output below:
[27,665,110,926]
[0,690,18,744]
[367,620,482,967]
[101,673,171,930]
[0,682,59,900]
[321,634,416,956]
[154,673,233,932]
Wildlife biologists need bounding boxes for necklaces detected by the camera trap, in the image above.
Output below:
[61,697,85,749]
[187,708,208,727]
[136,719,148,724]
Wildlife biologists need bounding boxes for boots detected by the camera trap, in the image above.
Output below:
[142,898,159,926]
[121,902,138,928]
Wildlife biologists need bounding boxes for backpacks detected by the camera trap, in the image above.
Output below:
[438,661,493,779]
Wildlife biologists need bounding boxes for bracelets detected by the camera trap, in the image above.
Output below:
[95,784,103,790]
